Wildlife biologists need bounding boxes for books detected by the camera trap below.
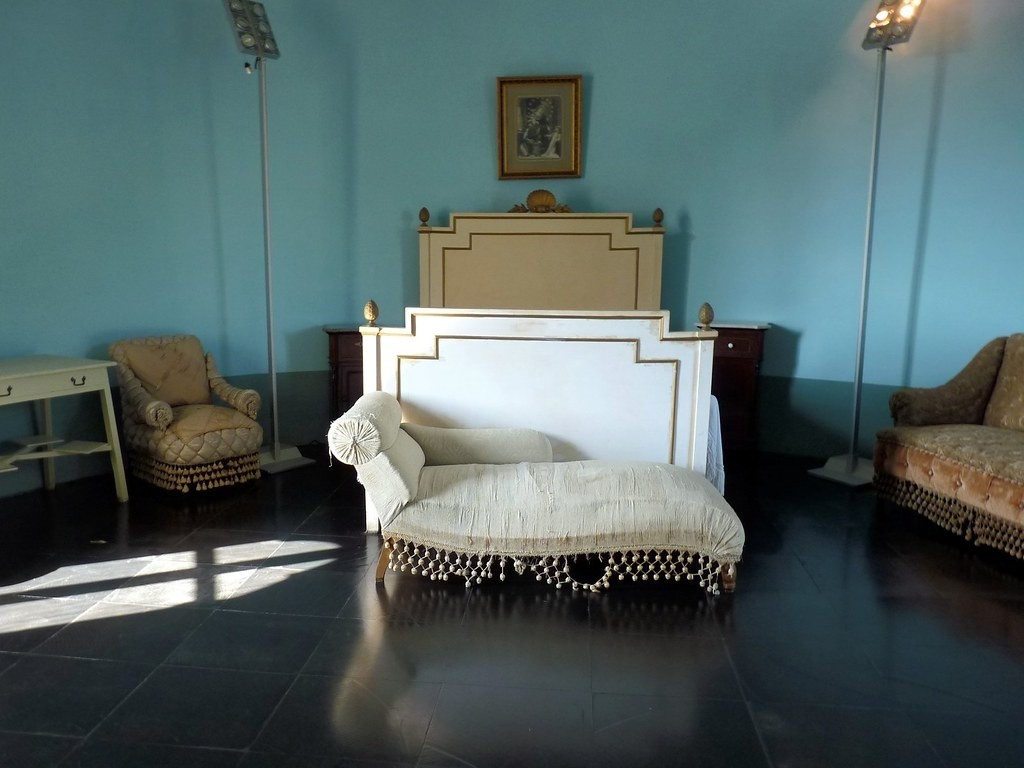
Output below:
[55,441,107,454]
[0,435,64,473]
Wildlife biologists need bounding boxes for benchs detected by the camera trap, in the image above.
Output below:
[327,392,745,604]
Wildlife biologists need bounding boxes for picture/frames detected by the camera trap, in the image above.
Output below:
[496,74,583,181]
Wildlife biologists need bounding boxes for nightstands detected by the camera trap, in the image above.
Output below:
[695,323,767,463]
[323,326,363,421]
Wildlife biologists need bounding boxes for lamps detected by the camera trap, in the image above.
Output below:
[807,0,926,487]
[224,0,316,474]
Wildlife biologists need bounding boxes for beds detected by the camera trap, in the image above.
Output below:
[358,189,717,530]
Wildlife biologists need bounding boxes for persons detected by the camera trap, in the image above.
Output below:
[523,108,559,158]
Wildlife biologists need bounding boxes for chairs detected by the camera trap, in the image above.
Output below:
[107,334,263,505]
[873,333,1024,560]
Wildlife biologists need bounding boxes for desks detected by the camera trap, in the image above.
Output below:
[1,354,129,502]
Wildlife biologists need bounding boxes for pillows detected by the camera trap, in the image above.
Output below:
[399,423,553,466]
